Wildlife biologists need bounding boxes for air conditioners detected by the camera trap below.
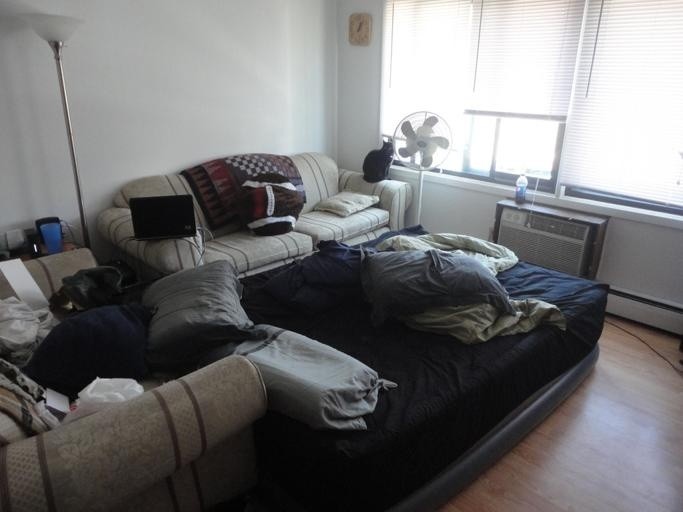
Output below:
[493,199,609,282]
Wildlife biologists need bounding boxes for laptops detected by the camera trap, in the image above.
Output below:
[129,194,197,241]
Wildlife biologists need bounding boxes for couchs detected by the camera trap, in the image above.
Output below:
[0,247,268,512]
[96,151,413,280]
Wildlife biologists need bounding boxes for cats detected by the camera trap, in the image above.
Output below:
[361,139,395,183]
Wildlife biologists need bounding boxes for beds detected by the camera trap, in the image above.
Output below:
[177,225,609,512]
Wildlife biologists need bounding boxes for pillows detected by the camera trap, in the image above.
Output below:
[0,258,50,313]
[313,191,380,218]
[359,244,517,321]
[141,259,268,374]
[23,302,157,399]
[196,323,399,431]
[260,240,394,317]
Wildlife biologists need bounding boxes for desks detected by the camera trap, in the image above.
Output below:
[0,235,83,265]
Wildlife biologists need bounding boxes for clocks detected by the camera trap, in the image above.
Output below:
[348,13,370,44]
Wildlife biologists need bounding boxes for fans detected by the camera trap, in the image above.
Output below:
[392,111,453,228]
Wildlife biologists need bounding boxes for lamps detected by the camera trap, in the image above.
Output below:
[26,12,92,256]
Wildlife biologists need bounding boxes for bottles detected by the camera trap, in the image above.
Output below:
[515,171,529,205]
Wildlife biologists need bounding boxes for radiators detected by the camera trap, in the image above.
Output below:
[605,284,683,336]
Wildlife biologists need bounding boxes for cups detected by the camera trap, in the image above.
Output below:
[40,222,64,255]
